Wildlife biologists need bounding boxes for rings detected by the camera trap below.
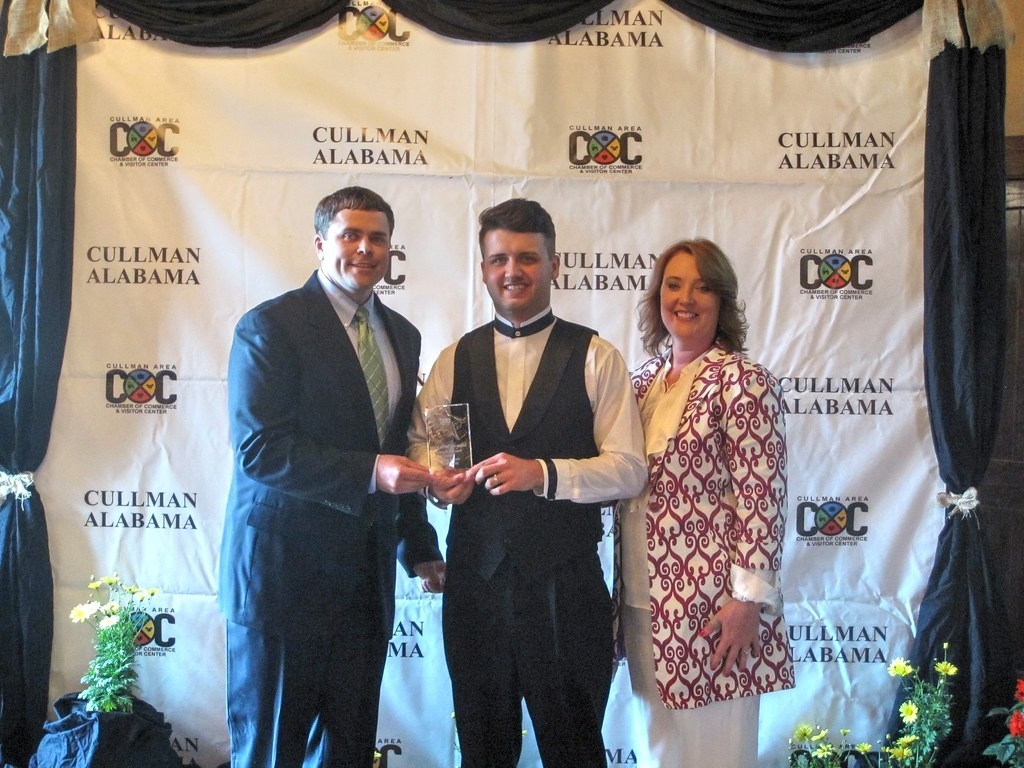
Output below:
[742,648,752,655]
[493,474,498,482]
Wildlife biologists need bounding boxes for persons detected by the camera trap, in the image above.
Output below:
[403,199,648,768]
[600,238,793,768]
[216,186,445,768]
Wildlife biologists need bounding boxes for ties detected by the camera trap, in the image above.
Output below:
[355,306,389,448]
